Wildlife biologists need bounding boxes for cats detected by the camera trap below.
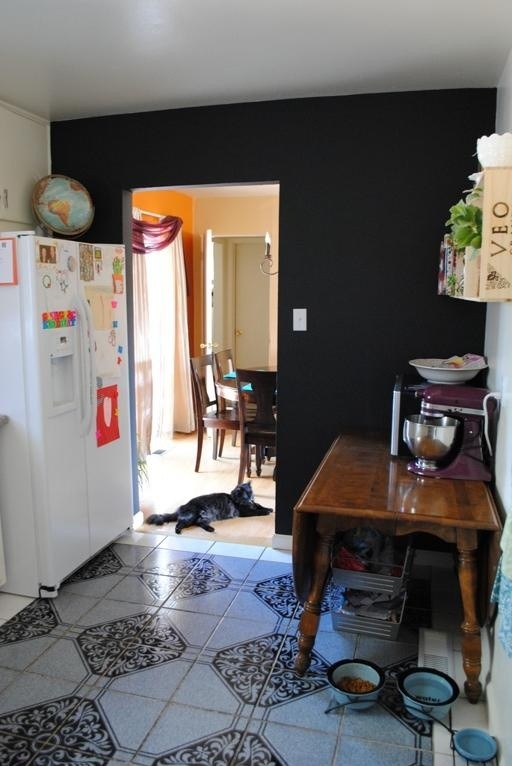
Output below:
[144,480,274,535]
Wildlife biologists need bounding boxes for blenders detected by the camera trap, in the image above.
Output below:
[403,386,496,483]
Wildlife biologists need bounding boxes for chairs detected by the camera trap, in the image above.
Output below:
[187,355,240,470]
[235,368,276,486]
[213,349,237,411]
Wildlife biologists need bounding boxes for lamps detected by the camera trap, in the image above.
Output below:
[258,230,279,276]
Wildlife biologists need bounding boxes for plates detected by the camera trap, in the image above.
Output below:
[454,728,497,762]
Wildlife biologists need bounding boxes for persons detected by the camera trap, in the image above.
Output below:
[40,247,48,263]
[49,247,55,263]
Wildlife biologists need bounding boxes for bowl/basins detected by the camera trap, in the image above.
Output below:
[329,659,385,712]
[408,354,489,385]
[396,666,463,719]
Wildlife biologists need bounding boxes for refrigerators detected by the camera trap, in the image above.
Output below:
[0,229,134,597]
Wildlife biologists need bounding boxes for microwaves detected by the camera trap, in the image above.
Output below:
[390,372,486,458]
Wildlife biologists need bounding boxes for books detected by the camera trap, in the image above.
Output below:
[435,233,465,296]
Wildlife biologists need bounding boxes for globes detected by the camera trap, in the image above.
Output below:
[31,173,95,240]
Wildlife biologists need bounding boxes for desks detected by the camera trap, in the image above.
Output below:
[293,423,507,703]
[215,377,275,463]
